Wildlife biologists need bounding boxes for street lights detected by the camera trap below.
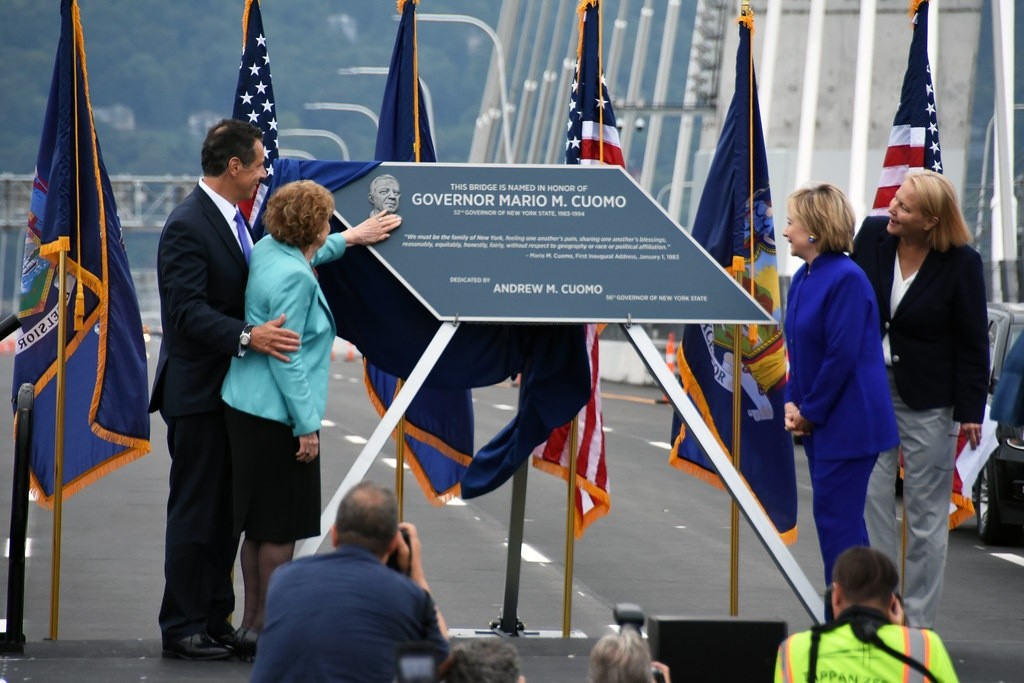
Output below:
[338,67,437,161]
[279,127,351,162]
[391,13,514,164]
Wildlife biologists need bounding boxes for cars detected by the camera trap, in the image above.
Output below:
[974,302,1024,543]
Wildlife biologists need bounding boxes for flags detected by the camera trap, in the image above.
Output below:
[212,1,974,542]
[13,0,150,506]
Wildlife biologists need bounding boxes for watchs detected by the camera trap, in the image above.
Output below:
[239,323,254,350]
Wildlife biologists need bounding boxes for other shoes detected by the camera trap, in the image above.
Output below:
[231,628,260,662]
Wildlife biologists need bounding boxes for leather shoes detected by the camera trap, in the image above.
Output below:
[163,630,235,659]
[206,623,236,646]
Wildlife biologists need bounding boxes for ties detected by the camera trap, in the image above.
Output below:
[233,210,252,268]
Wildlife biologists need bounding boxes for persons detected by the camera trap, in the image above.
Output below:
[367,175,401,218]
[249,481,527,682]
[588,631,670,683]
[145,119,401,661]
[781,169,990,630]
[774,545,960,683]
